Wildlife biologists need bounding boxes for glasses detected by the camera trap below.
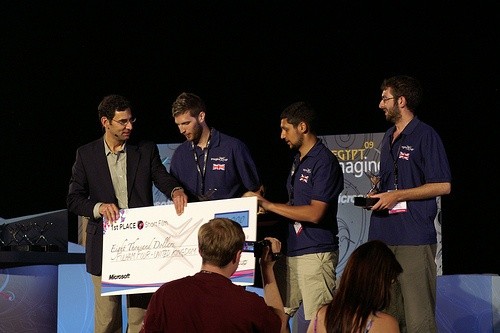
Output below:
[383,97,398,102]
[112,117,136,125]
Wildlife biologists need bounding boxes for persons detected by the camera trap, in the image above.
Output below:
[169,91,267,215]
[67,93,187,333]
[138,218,290,333]
[306,239,403,333]
[241,102,345,333]
[368,69,450,333]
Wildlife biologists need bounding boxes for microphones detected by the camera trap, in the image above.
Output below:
[108,127,118,137]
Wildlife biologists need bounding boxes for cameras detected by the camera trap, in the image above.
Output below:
[242,240,272,257]
